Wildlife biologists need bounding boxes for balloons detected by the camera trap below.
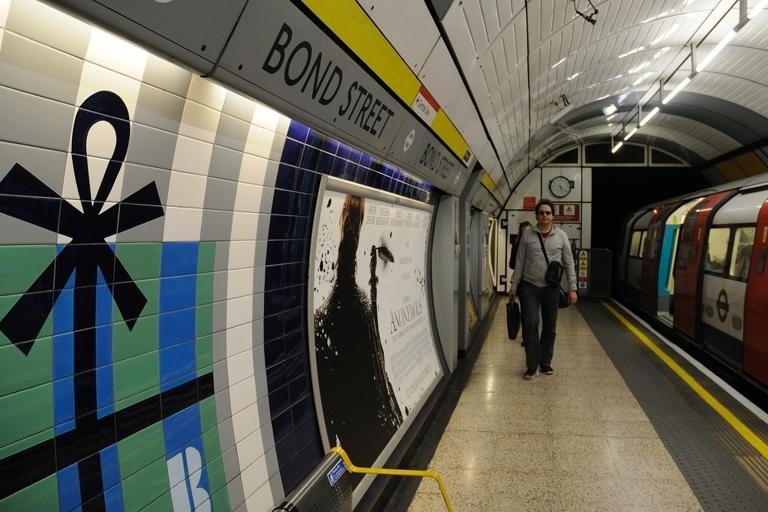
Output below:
[549,176,571,198]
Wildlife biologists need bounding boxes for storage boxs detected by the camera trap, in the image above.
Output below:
[272,447,454,512]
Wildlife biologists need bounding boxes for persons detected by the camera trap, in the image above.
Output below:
[508,199,578,380]
[509,221,533,347]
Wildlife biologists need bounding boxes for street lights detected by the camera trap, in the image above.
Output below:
[507,302,519,340]
[545,262,563,285]
[559,287,570,308]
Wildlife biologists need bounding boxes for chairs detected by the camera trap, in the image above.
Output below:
[541,364,554,375]
[524,365,539,379]
